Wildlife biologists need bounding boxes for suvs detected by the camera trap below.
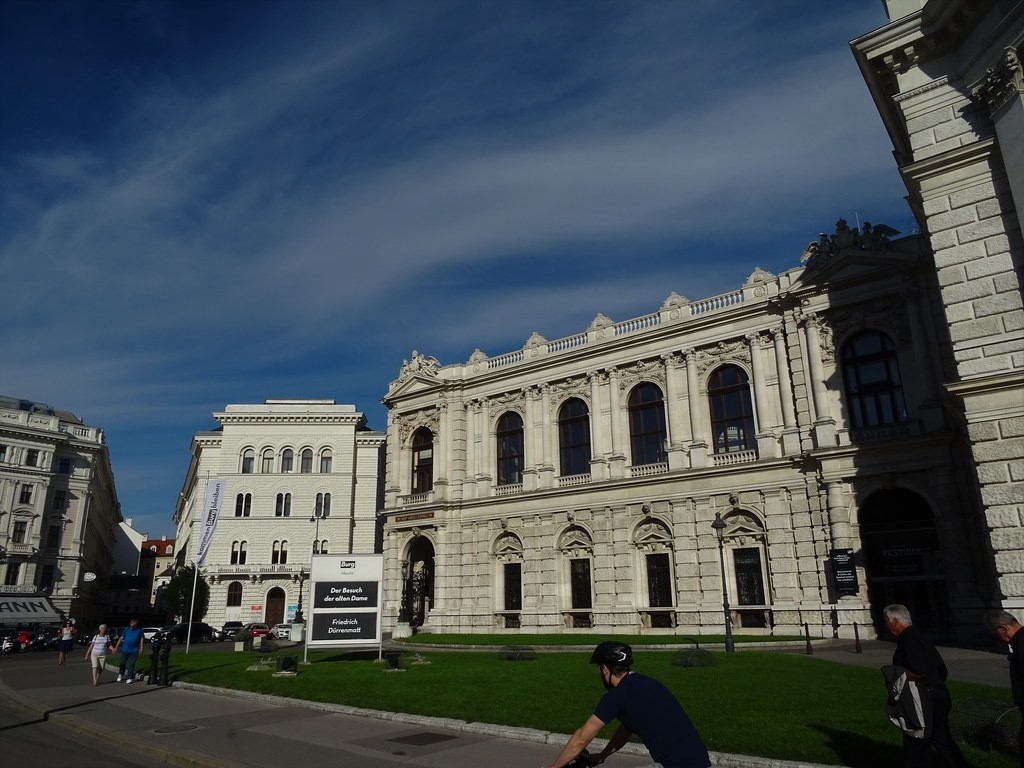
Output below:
[149,622,213,646]
[221,621,244,642]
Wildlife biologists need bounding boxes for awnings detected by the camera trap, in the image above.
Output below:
[157,564,173,579]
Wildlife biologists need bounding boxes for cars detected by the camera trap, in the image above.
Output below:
[249,623,270,640]
[0,625,63,655]
[141,627,164,644]
[270,623,293,640]
[76,626,125,646]
[211,627,223,643]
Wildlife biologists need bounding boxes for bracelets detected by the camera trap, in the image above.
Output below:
[141,647,143,648]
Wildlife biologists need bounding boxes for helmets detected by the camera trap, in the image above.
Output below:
[590,641,633,666]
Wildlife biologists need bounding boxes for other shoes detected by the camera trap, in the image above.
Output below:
[59,662,66,665]
[126,679,133,684]
[93,681,99,686]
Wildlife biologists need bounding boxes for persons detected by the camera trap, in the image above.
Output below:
[59,620,77,665]
[84,624,118,686]
[542,641,713,768]
[882,603,970,768]
[984,609,1024,768]
[113,618,145,684]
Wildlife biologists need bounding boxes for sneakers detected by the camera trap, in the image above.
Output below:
[117,674,122,682]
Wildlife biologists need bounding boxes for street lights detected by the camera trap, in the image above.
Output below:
[710,511,735,653]
[392,560,413,639]
[291,568,308,641]
[309,504,327,553]
